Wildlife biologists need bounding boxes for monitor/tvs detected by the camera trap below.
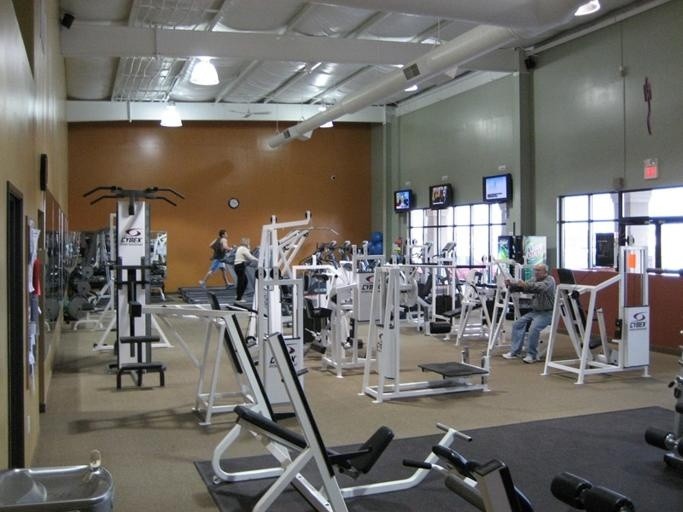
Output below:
[429,183,453,210]
[483,173,513,203]
[394,190,412,213]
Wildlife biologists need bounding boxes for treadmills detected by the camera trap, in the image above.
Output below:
[178,246,259,303]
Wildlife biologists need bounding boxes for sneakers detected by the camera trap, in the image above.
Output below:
[198,280,206,289]
[225,283,235,289]
[234,298,246,303]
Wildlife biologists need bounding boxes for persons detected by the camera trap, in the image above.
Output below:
[232,237,259,302]
[195,228,234,290]
[500,263,556,361]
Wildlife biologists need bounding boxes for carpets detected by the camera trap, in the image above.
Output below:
[188,406,683,512]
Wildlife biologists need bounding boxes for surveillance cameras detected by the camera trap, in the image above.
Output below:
[331,175,336,181]
